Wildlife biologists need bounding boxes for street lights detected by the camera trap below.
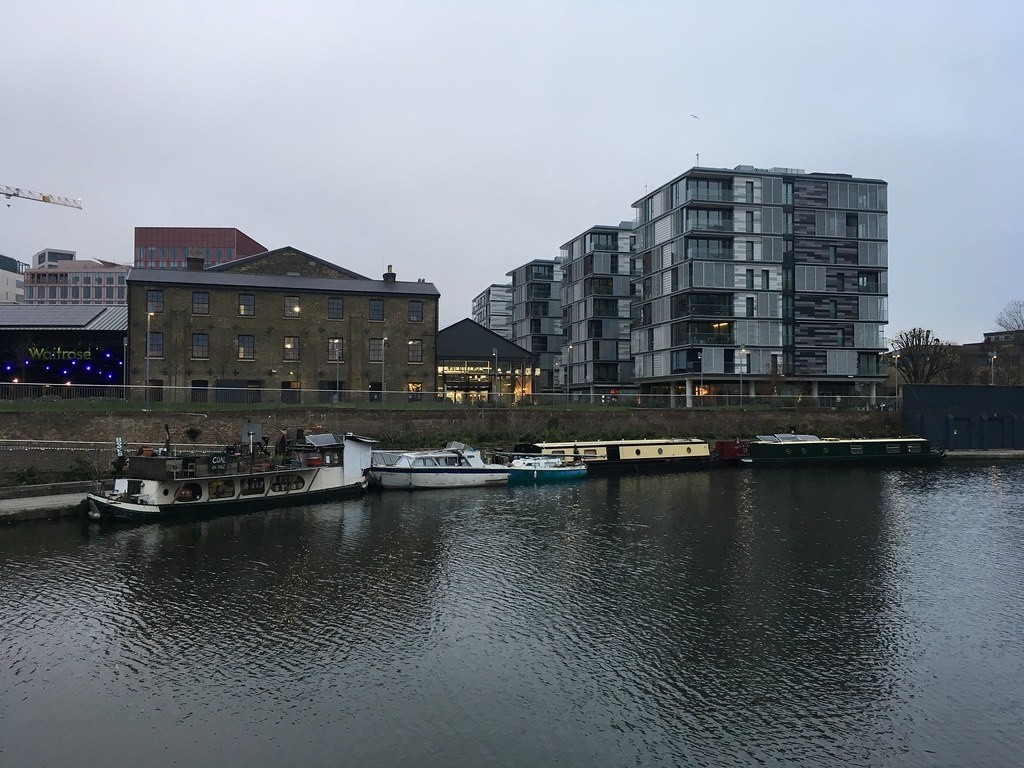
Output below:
[382,338,387,403]
[892,351,901,411]
[567,341,572,403]
[123,337,127,399]
[493,348,498,407]
[146,313,155,410]
[739,344,746,405]
[988,351,997,385]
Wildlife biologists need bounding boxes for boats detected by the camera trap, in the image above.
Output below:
[507,457,588,482]
[86,421,380,522]
[364,441,511,488]
[494,439,723,473]
[740,433,947,466]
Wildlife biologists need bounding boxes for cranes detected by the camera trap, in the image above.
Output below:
[0,185,82,210]
